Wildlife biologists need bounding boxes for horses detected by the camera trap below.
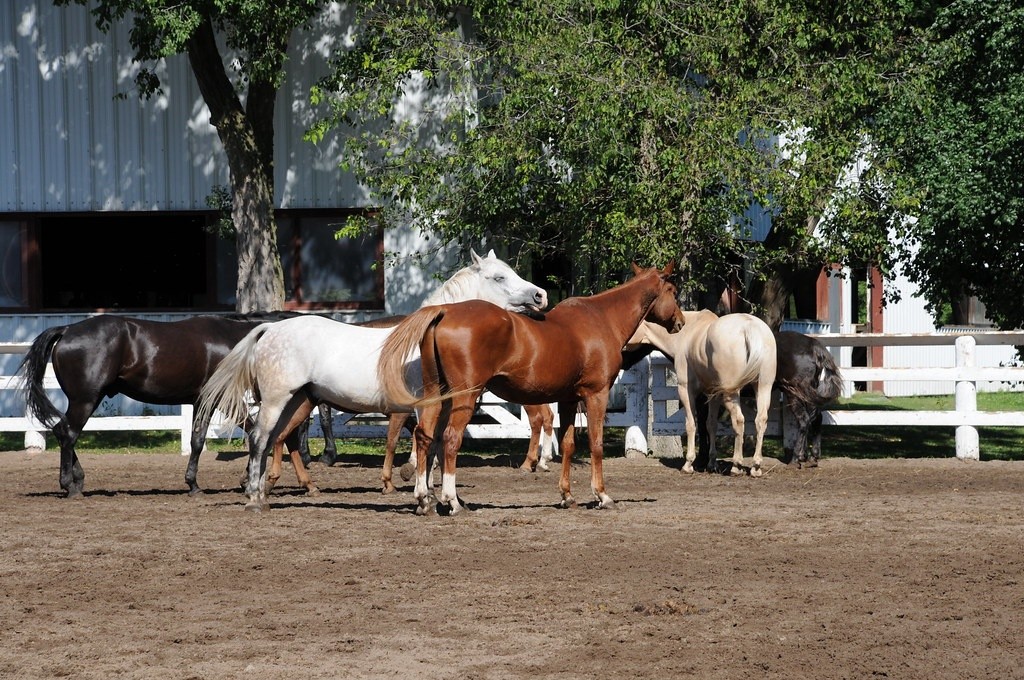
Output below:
[22,248,843,518]
